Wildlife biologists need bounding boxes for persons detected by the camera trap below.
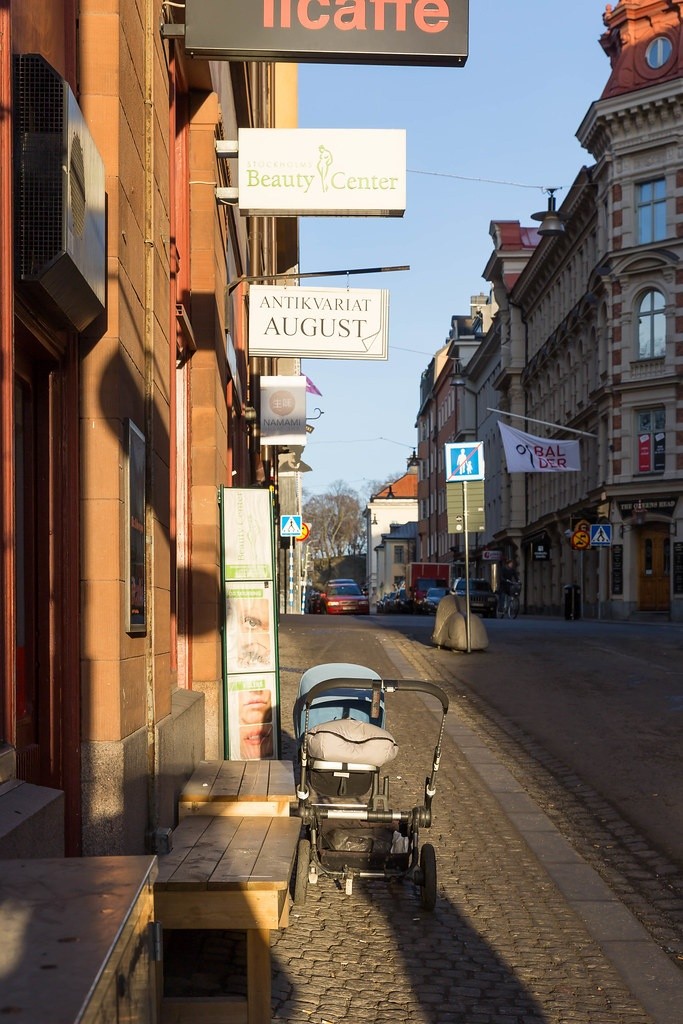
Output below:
[497,558,520,619]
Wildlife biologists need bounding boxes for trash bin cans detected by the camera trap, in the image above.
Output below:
[564,584,581,621]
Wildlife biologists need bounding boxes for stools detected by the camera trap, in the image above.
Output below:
[153,816,303,1024]
[177,758,300,816]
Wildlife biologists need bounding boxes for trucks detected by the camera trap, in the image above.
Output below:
[405,562,450,614]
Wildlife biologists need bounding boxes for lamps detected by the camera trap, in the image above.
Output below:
[245,403,258,435]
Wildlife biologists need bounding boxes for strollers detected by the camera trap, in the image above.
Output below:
[290,662,448,913]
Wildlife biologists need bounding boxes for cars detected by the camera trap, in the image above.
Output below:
[306,579,360,614]
[375,588,411,614]
[319,583,369,616]
[422,588,449,615]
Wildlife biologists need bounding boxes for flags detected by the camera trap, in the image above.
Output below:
[497,419,582,473]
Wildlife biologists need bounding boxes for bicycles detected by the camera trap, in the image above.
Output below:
[495,577,522,619]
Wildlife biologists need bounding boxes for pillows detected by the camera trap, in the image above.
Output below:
[300,719,399,768]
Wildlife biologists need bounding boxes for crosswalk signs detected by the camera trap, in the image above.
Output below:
[590,523,613,547]
[281,514,303,537]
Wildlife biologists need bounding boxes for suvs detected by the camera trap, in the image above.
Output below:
[448,576,499,619]
